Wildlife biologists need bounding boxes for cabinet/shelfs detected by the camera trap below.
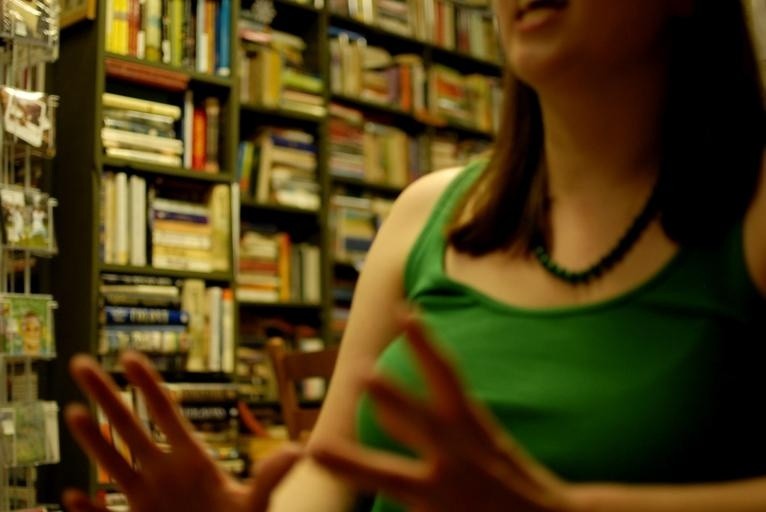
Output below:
[48,0,240,512]
[229,0,332,439]
[326,1,508,341]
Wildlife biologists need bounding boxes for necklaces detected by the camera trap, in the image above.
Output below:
[531,186,655,291]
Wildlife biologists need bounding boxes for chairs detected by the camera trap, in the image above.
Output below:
[264,337,344,444]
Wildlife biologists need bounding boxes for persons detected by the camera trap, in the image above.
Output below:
[8,203,27,243]
[0,304,22,349]
[65,1,766,512]
[28,203,51,246]
[0,201,14,245]
[20,311,48,357]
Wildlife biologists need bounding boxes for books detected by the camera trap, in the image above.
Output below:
[327,30,506,136]
[327,193,394,344]
[99,169,232,277]
[98,272,236,373]
[327,2,507,66]
[101,60,225,175]
[238,1,325,117]
[96,378,271,511]
[239,231,323,304]
[236,316,328,404]
[105,2,231,77]
[324,104,483,189]
[240,409,293,481]
[237,127,325,212]
